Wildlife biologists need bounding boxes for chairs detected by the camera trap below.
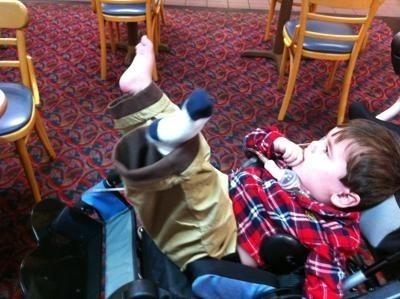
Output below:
[0,0,55,204]
[276,0,385,126]
[91,0,160,83]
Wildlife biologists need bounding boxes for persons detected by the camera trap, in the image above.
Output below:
[107,34,400,299]
